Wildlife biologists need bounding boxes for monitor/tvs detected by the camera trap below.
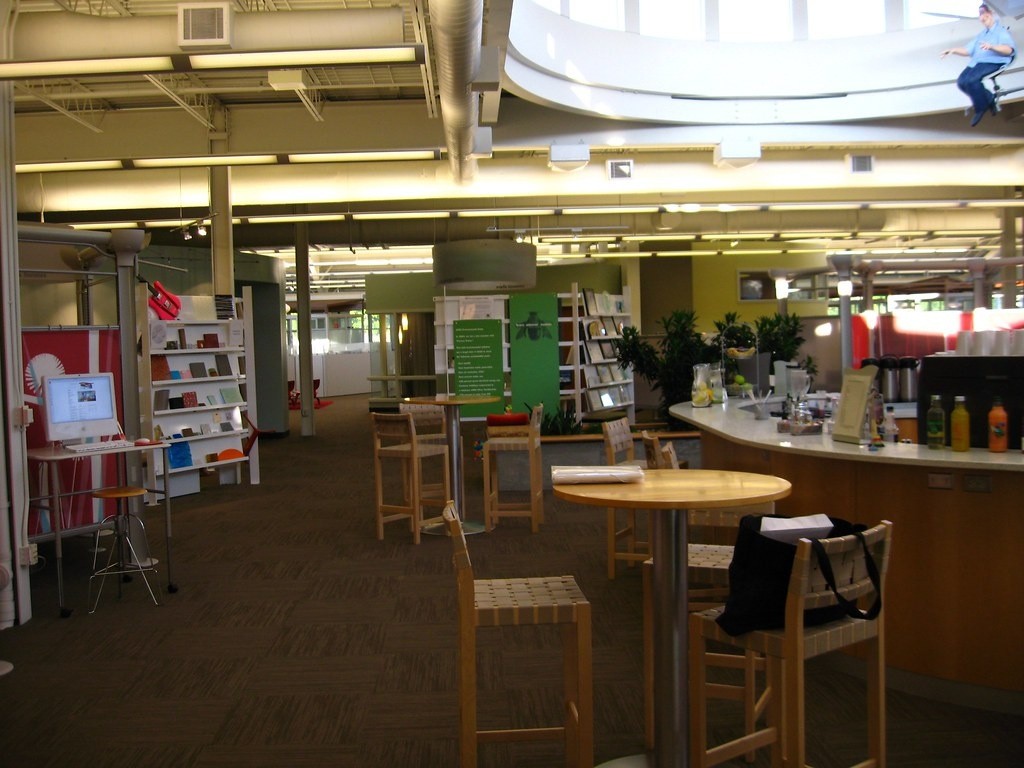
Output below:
[916,355,1024,451]
[41,372,118,445]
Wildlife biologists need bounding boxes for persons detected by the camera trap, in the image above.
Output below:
[940,3,1016,127]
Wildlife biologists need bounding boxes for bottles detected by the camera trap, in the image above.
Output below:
[949,396,971,451]
[884,406,897,430]
[927,394,947,451]
[987,396,1009,453]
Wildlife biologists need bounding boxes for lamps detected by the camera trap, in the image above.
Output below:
[0,43,425,80]
[14,147,442,174]
[712,137,760,170]
[69,199,1024,258]
[548,144,591,172]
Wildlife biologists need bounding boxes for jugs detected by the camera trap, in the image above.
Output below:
[773,360,801,396]
[861,355,920,403]
[786,364,811,400]
[691,363,713,408]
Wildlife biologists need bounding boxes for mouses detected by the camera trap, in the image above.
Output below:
[135,438,150,444]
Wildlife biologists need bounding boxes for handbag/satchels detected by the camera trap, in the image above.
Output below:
[715,510,883,638]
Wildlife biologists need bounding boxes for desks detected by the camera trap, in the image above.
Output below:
[405,395,501,536]
[28,442,178,618]
[553,469,792,768]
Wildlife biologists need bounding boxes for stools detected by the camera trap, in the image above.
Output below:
[87,486,164,614]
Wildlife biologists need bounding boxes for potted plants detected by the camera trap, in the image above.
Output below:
[613,308,817,430]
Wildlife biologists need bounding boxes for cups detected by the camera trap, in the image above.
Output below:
[955,331,1024,358]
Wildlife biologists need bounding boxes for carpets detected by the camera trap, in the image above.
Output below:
[288,400,333,409]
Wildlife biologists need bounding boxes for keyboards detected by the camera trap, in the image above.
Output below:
[65,439,134,453]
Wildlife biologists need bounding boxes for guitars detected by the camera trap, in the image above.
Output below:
[136,274,182,320]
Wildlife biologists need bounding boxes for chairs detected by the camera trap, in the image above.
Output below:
[370,402,546,544]
[288,379,320,405]
[963,24,1024,118]
[442,500,595,768]
[601,417,894,768]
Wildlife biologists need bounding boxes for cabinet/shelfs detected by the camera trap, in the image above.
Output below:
[135,282,260,506]
[432,283,636,425]
